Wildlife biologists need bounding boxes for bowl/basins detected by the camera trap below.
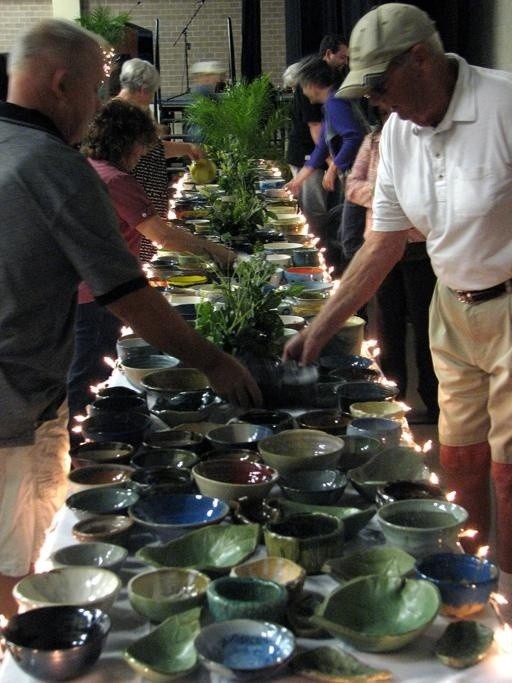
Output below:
[305,574,441,653]
[50,164,474,550]
[193,617,295,679]
[10,566,121,613]
[290,647,393,681]
[414,552,497,617]
[48,544,127,573]
[230,556,306,600]
[204,575,287,622]
[5,605,112,678]
[431,619,496,668]
[128,567,213,623]
[321,545,417,585]
[122,607,200,681]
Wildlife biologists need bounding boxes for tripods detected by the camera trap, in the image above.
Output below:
[167,2,205,100]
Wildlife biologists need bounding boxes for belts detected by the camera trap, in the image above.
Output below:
[445,279,512,306]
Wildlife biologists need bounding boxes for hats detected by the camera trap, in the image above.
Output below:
[332,3,437,100]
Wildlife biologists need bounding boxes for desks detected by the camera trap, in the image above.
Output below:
[6,144,512,682]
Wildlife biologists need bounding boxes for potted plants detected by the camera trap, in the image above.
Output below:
[181,72,293,401]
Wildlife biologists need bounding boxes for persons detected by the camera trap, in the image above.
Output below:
[112,57,208,266]
[64,96,235,452]
[0,13,263,635]
[272,33,438,427]
[277,3,512,582]
